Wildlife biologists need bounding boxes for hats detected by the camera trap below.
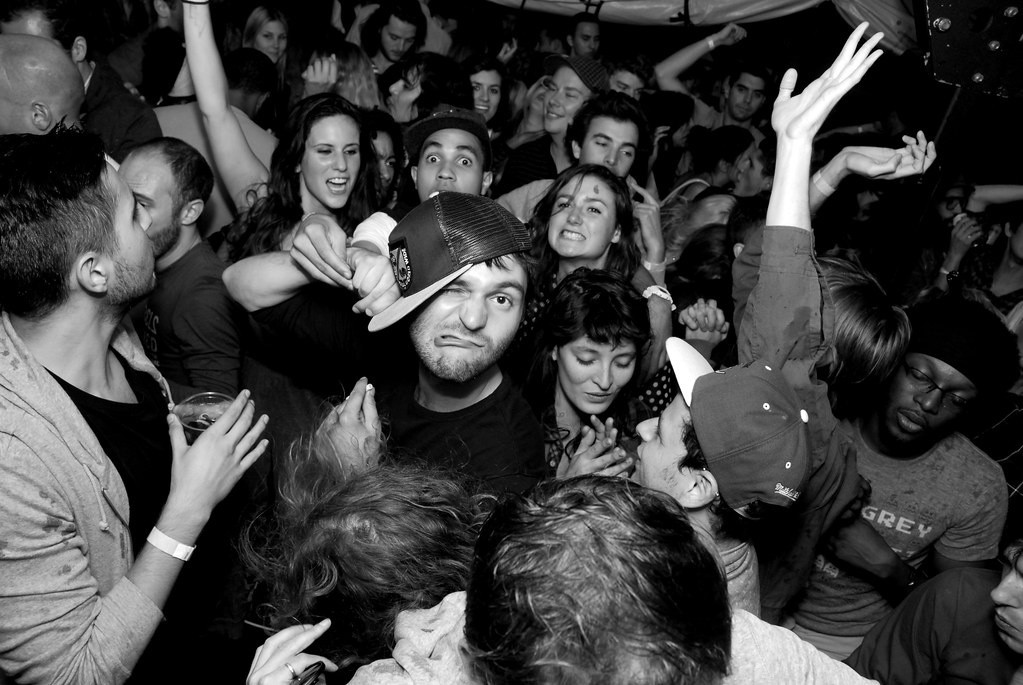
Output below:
[402,107,491,168]
[905,291,1023,409]
[367,191,532,332]
[666,336,809,518]
[544,53,609,93]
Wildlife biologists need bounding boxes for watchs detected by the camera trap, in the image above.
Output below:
[642,285,677,311]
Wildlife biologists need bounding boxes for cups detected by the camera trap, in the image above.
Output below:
[173,391,235,448]
[952,213,976,241]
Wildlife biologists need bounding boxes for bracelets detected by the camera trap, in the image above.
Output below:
[940,267,949,274]
[812,170,836,197]
[147,526,197,561]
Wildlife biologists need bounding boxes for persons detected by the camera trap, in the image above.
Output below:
[0,0,1023,685]
[840,496,1023,685]
[246,474,881,685]
[0,114,269,685]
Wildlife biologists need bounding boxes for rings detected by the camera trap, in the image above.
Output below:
[286,663,296,677]
